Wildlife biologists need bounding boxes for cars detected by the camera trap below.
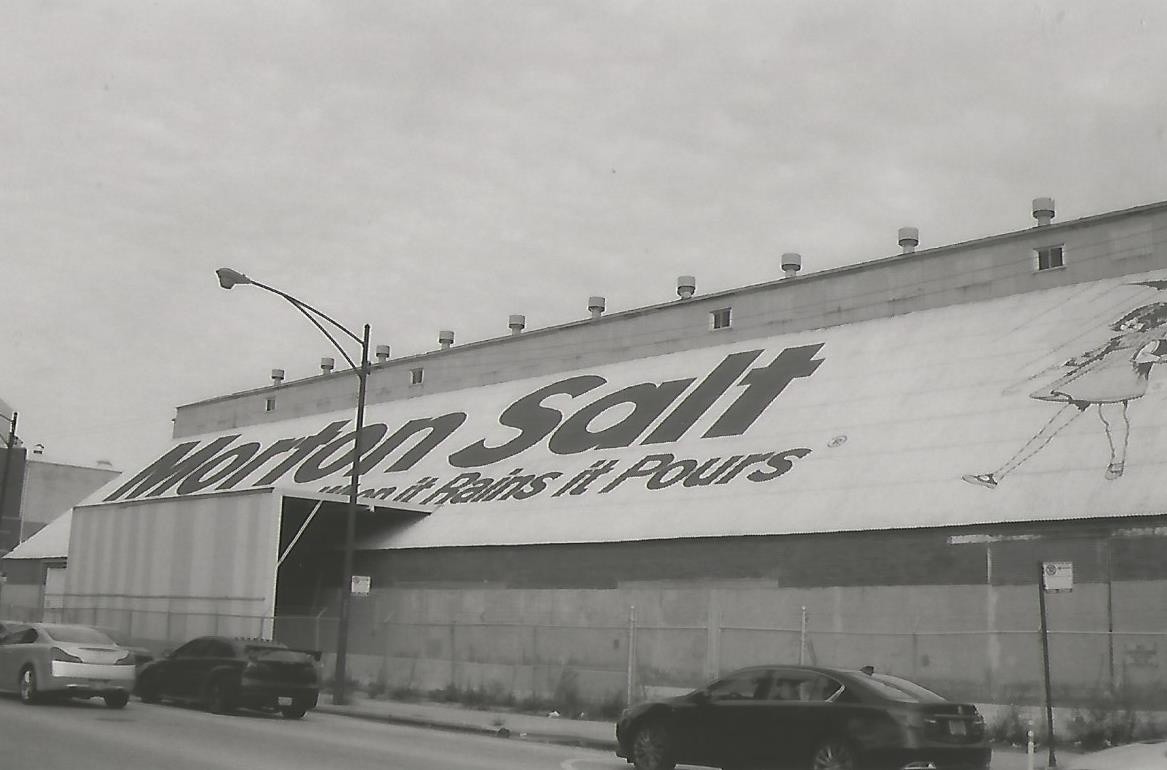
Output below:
[135,634,321,718]
[0,622,138,708]
[614,661,996,769]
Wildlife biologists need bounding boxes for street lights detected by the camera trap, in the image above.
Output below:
[216,267,371,713]
